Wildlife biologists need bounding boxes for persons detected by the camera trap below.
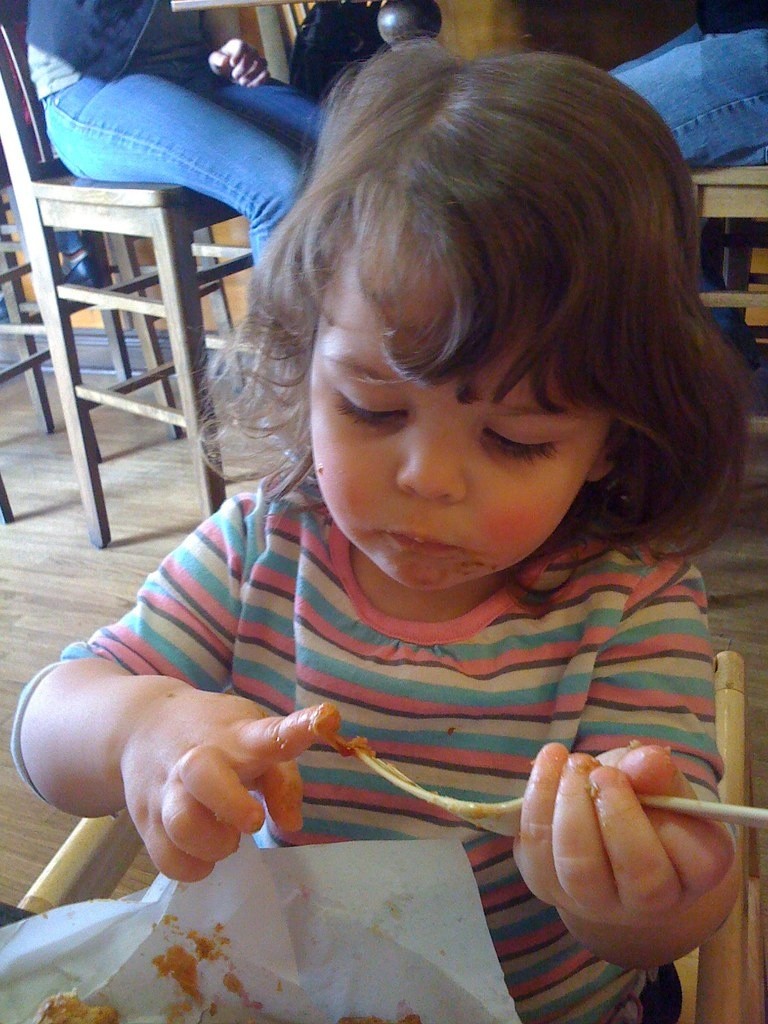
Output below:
[27,0,327,272]
[10,38,743,1024]
[0,0,103,291]
[609,0,767,372]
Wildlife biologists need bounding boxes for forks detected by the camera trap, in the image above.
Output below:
[350,742,768,836]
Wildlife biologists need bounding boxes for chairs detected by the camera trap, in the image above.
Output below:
[14,650,767,1024]
[0,0,317,562]
[686,165,768,338]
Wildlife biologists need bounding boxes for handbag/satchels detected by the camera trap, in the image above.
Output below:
[291,0,389,98]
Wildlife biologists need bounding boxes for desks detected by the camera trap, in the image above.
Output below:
[171,0,443,50]
